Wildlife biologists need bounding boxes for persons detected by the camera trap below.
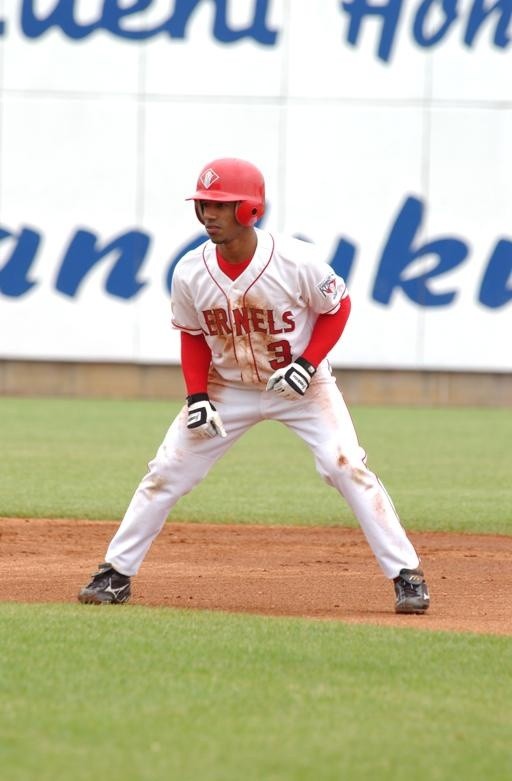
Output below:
[74,155,433,616]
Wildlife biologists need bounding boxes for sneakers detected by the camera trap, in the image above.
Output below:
[76,561,132,607]
[390,567,430,615]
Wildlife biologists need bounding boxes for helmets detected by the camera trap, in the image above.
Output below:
[184,158,267,232]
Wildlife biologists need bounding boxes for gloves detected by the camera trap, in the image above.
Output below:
[184,393,227,440]
[265,356,316,401]
